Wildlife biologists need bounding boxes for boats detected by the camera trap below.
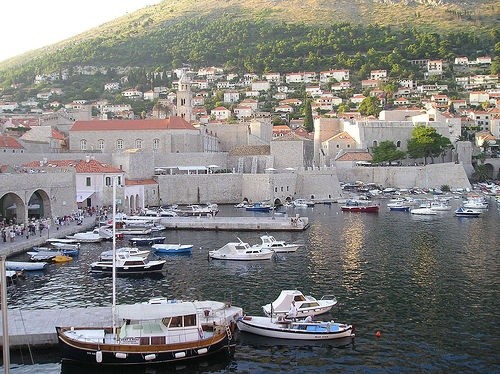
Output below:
[5,260,48,271]
[84,296,231,315]
[86,251,166,276]
[338,182,500,210]
[250,234,303,253]
[150,243,194,255]
[128,236,166,247]
[410,208,437,215]
[340,202,380,214]
[236,314,356,340]
[55,307,236,374]
[389,205,410,212]
[23,180,220,258]
[236,198,318,216]
[454,207,482,218]
[262,289,338,319]
[208,236,275,261]
